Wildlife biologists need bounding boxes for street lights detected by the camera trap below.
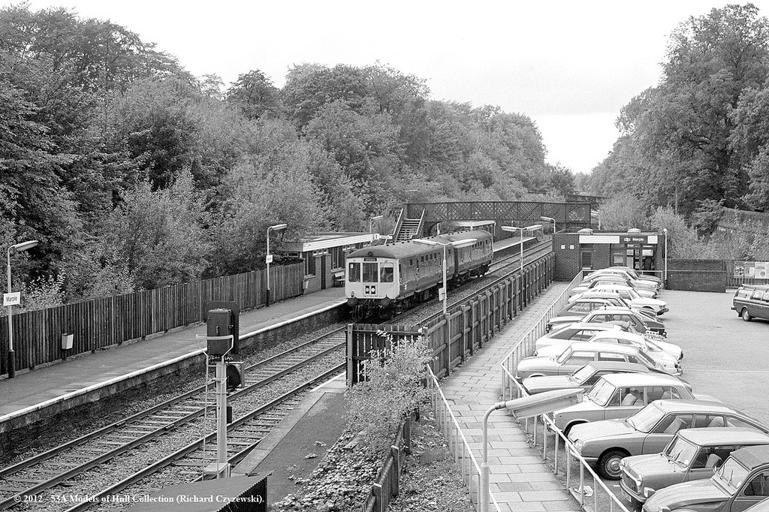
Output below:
[478,386,588,512]
[369,215,384,234]
[265,222,288,306]
[539,215,557,239]
[5,238,39,380]
[662,228,669,288]
[500,225,544,274]
[412,237,479,319]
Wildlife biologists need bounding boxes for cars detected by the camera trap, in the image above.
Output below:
[514,266,698,383]
[521,360,694,396]
[730,282,761,322]
[565,398,769,481]
[734,261,754,276]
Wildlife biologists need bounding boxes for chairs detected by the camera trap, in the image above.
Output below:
[622,390,640,406]
[664,417,687,434]
[634,392,649,406]
[704,454,723,468]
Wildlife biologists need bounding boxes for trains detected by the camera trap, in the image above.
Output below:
[343,228,495,323]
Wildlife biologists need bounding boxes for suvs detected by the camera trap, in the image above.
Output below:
[618,428,768,509]
[640,445,768,512]
[540,373,699,446]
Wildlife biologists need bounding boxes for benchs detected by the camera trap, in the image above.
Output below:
[333,271,345,286]
[661,390,679,399]
[708,417,736,427]
